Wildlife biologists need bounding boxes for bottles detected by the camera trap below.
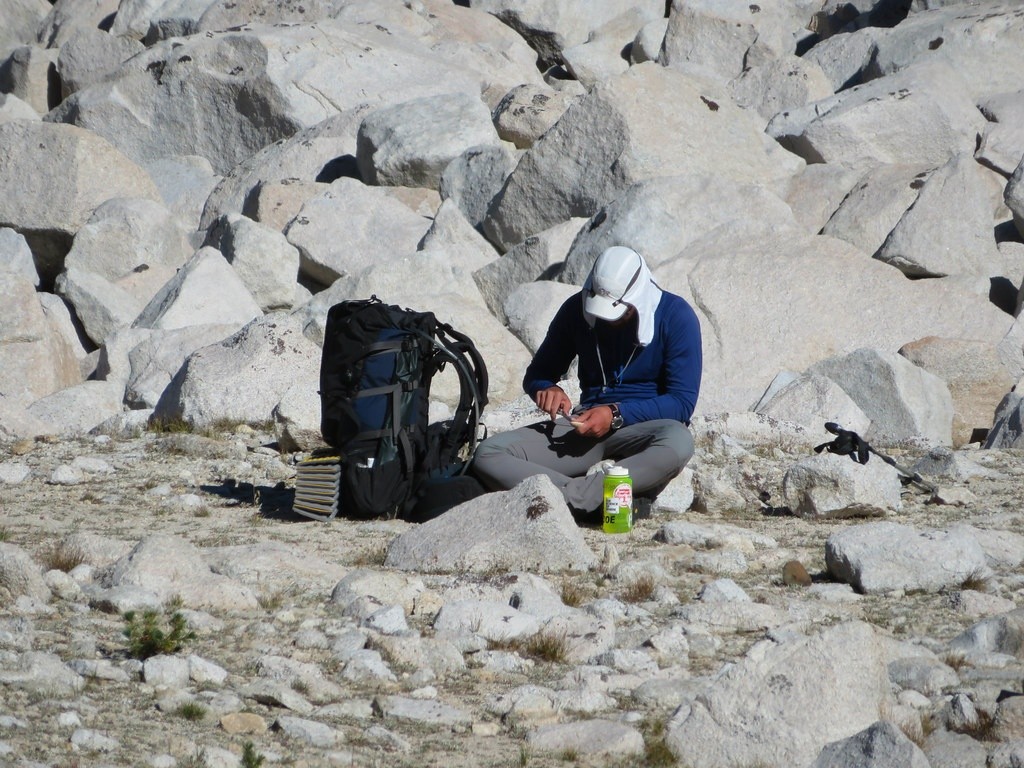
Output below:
[601,461,632,534]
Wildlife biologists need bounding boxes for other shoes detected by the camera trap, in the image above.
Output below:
[633,497,652,519]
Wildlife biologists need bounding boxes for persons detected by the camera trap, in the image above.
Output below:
[473,245,703,524]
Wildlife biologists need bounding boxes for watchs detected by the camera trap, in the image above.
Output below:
[607,404,624,432]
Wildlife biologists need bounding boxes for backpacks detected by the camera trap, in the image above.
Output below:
[318,297,488,520]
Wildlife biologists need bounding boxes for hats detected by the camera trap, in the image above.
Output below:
[585,246,640,321]
